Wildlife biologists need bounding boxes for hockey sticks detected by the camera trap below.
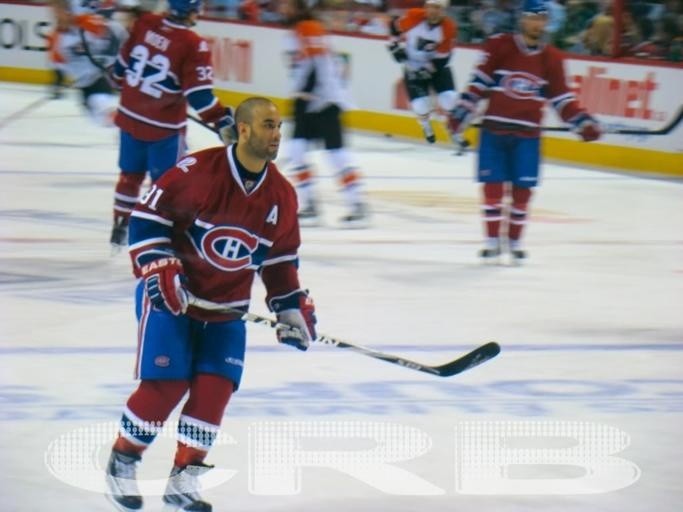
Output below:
[469,104,682,134]
[180,293,500,378]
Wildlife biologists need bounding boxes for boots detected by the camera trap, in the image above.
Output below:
[294,197,368,221]
[478,243,504,258]
[104,446,144,509]
[508,236,529,260]
[421,122,440,145]
[162,459,216,511]
[108,222,130,248]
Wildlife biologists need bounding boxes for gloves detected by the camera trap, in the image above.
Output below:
[216,107,242,147]
[574,115,600,142]
[265,290,317,350]
[139,259,191,316]
[446,93,480,131]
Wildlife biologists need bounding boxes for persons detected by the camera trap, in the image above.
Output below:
[275,0,373,223]
[387,0,473,154]
[43,0,138,130]
[187,1,682,67]
[105,96,317,512]
[445,0,601,267]
[108,0,240,250]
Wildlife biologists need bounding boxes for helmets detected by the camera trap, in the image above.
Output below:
[166,1,201,13]
[520,0,549,16]
